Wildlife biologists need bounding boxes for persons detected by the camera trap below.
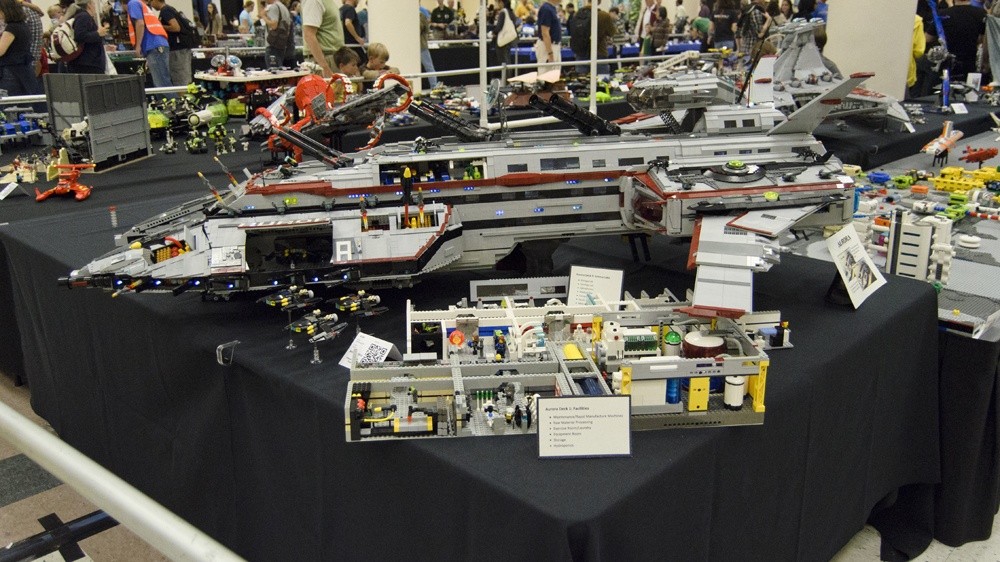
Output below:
[0,0,1000,99]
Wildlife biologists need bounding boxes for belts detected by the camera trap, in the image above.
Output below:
[302,48,337,59]
[539,37,561,46]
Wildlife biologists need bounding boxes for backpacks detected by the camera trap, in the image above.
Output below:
[570,8,593,57]
[48,18,85,64]
[166,6,202,49]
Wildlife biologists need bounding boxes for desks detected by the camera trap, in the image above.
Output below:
[3,120,1000,560]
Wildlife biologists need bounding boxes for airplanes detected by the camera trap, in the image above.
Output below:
[55,49,999,311]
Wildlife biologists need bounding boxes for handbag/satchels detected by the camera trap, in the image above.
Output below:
[267,19,290,52]
[104,50,119,75]
[496,8,518,48]
